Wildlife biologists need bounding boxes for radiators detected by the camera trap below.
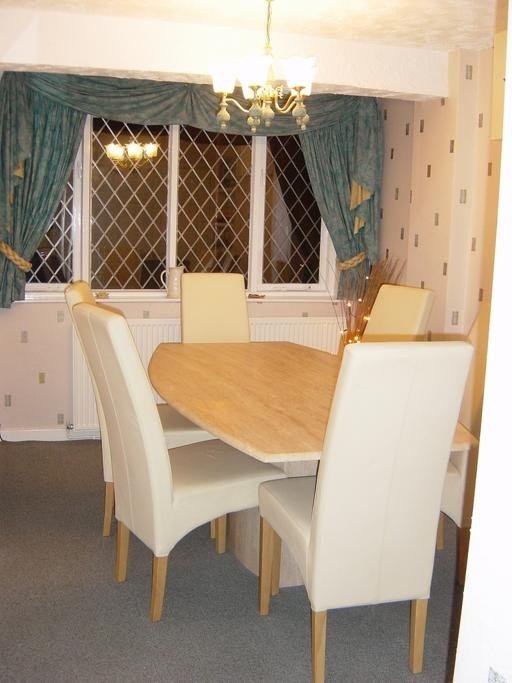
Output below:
[71,316,346,429]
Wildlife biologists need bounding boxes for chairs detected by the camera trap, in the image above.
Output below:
[73,304,285,620]
[180,272,250,343]
[337,284,435,342]
[258,340,473,682]
[64,281,217,539]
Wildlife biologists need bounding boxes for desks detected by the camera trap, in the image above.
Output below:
[147,338,480,588]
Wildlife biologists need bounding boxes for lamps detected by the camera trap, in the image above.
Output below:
[104,136,161,169]
[212,1,317,133]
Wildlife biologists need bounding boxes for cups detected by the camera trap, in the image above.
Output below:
[160,266,183,297]
